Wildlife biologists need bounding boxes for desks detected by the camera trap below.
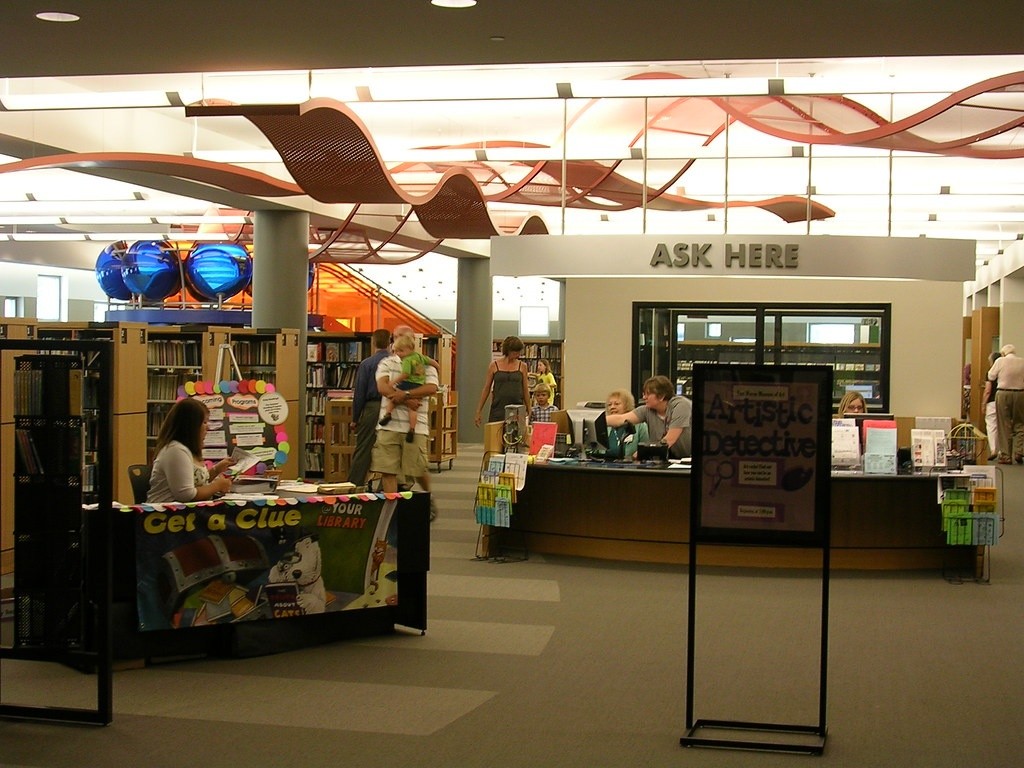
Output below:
[79,490,431,670]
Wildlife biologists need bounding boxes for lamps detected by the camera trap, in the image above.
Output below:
[0,58,1024,243]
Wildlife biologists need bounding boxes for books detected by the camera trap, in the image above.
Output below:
[15,338,363,494]
[422,340,437,361]
[493,341,561,410]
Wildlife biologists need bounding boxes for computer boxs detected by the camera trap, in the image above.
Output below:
[504,405,528,455]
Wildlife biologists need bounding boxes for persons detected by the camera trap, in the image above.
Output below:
[604,376,692,459]
[980,344,1024,465]
[379,335,441,443]
[838,391,867,419]
[606,390,649,460]
[528,383,560,424]
[474,335,530,427]
[528,359,557,406]
[347,329,391,487]
[145,398,236,503]
[368,326,439,522]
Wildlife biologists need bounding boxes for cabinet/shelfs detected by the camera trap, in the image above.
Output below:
[673,342,882,405]
[492,337,564,411]
[1,316,459,576]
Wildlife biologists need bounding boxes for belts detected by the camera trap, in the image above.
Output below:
[998,389,1024,392]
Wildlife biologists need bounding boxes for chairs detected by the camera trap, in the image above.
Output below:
[128,463,153,504]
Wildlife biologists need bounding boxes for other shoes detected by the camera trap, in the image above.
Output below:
[379,413,391,426]
[999,458,1012,464]
[406,428,415,443]
[988,455,997,460]
[1015,454,1023,463]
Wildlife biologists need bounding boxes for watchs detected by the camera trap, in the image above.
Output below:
[405,390,411,399]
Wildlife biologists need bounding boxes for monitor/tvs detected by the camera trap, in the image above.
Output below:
[844,413,894,457]
[565,408,610,462]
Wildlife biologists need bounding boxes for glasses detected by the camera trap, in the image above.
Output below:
[847,405,863,411]
